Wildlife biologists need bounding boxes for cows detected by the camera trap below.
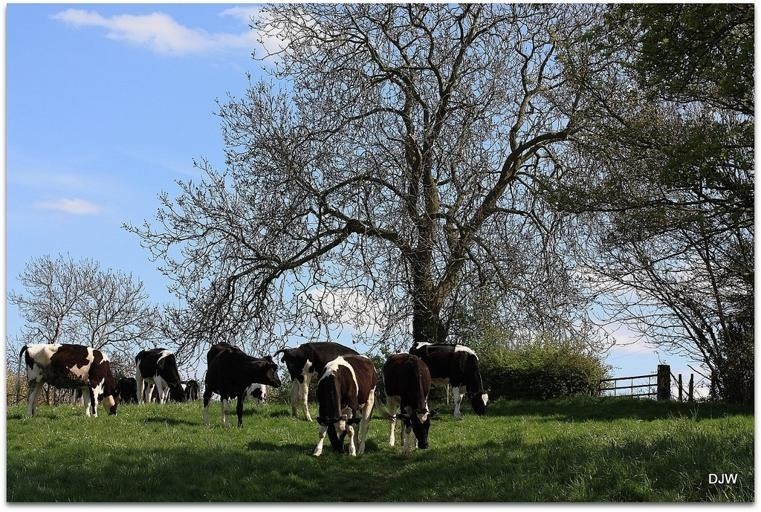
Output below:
[16,340,283,429]
[314,352,379,457]
[273,341,388,422]
[408,339,502,418]
[382,353,436,451]
